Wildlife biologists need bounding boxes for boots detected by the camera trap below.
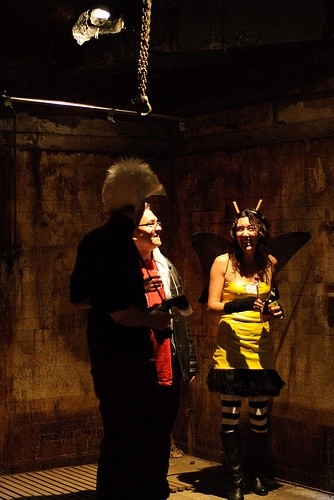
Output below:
[219,429,247,500]
[245,426,270,495]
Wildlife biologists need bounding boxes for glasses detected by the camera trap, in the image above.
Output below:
[136,220,163,226]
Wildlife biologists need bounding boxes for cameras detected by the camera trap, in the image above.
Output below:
[162,295,189,312]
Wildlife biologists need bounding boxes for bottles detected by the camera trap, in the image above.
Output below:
[263,288,276,321]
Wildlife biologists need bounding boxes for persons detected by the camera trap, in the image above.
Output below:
[207,199,284,499]
[131,203,193,500]
[69,157,176,500]
